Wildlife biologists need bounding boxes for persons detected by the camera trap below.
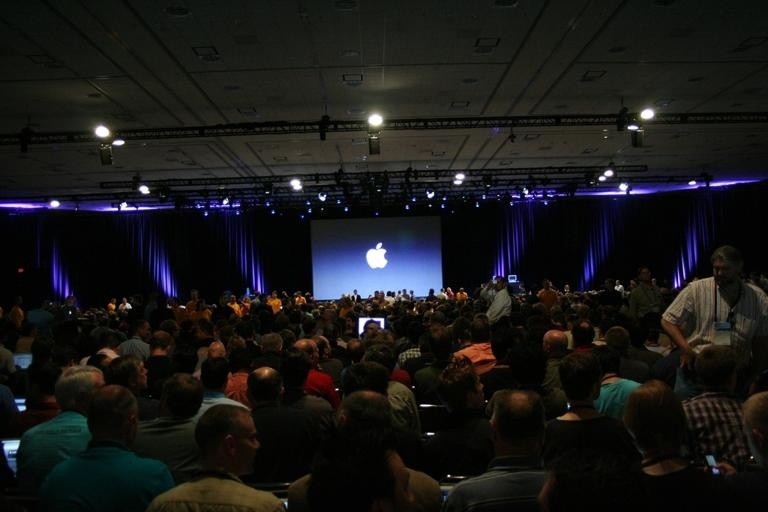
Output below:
[658,245,767,374]
[628,265,662,330]
[2,276,768,512]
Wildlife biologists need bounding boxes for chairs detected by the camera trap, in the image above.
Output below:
[0,365,768,512]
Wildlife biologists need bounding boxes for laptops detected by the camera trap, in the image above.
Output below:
[357,316,387,338]
[493,276,497,280]
[1,438,24,478]
[251,482,290,509]
[13,352,32,369]
[14,396,28,414]
[508,275,517,282]
[438,475,470,503]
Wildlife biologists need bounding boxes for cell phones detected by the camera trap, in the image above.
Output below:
[704,450,721,477]
